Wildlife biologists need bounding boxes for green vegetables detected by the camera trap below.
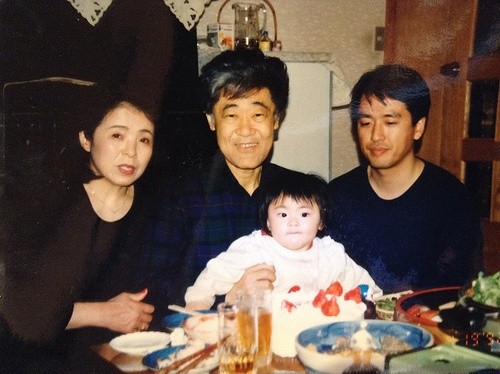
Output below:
[467,271,500,309]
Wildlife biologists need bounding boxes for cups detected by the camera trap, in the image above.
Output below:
[238,288,273,367]
[219,302,259,374]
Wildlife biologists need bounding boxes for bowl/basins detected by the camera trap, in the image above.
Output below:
[376,291,414,320]
[265,293,366,356]
[182,313,227,344]
[395,285,467,346]
[295,319,434,374]
[454,282,500,358]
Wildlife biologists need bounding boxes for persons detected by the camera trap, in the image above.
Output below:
[0,93,172,343]
[316,64,484,294]
[183,176,382,312]
[160,49,306,304]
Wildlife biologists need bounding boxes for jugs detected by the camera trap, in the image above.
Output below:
[232,2,268,43]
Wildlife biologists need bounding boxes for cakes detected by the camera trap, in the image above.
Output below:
[270,282,368,357]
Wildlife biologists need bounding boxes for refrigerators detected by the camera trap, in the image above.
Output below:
[197,45,332,184]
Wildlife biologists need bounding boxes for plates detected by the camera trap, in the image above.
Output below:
[159,310,218,329]
[384,343,500,374]
[109,331,170,355]
